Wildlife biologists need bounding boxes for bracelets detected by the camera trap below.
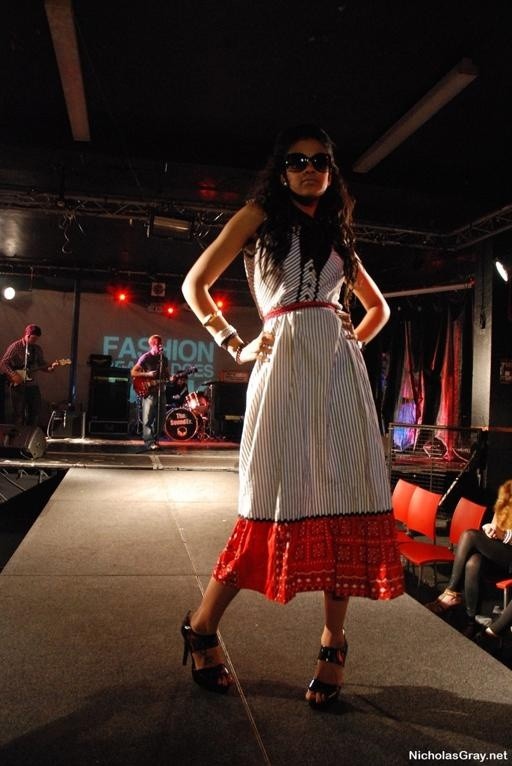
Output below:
[501,530,512,545]
[210,323,239,347]
[231,340,250,366]
[198,310,226,327]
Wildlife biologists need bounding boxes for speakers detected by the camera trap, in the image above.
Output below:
[0,423,47,459]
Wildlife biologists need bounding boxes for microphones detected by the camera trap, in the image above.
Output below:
[159,347,163,351]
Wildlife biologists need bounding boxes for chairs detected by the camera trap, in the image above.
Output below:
[391,478,512,609]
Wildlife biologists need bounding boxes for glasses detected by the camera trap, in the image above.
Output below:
[284,152,332,171]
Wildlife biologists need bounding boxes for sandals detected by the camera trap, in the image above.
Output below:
[424,589,462,613]
[462,615,496,645]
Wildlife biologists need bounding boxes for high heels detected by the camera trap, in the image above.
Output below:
[306,629,348,707]
[180,610,233,693]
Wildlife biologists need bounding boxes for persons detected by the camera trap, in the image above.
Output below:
[163,369,190,411]
[424,477,512,639]
[470,594,512,650]
[179,121,408,710]
[127,333,178,453]
[1,322,60,425]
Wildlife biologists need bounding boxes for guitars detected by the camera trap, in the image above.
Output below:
[133,366,198,398]
[3,358,72,387]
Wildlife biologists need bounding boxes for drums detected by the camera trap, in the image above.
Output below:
[183,392,209,417]
[162,406,199,441]
